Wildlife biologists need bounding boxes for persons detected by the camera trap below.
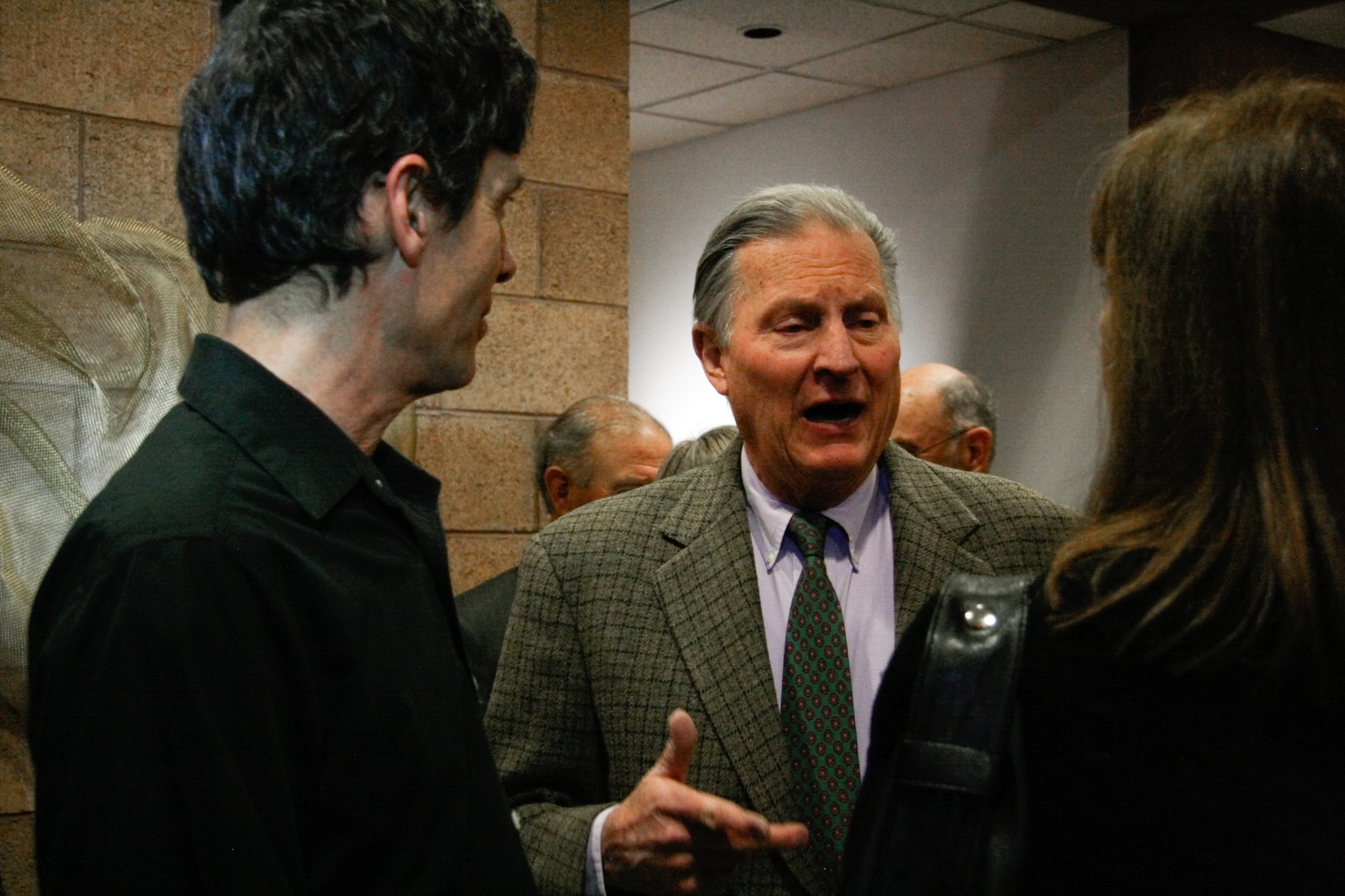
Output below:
[888,366,996,474]
[485,181,1088,896]
[25,0,536,896]
[453,397,675,718]
[654,425,739,481]
[824,65,1345,896]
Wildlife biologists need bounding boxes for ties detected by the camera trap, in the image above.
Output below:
[780,514,862,880]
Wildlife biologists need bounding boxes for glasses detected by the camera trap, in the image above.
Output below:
[895,429,970,456]
